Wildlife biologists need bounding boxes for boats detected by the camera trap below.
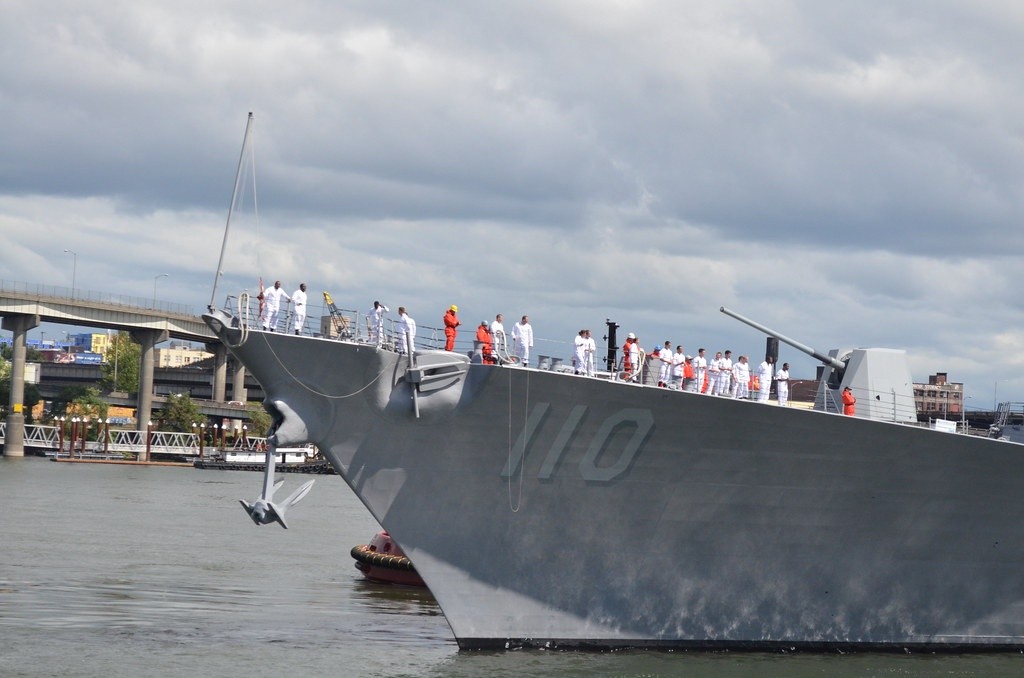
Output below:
[193,443,340,474]
[202,307,1023,651]
[350,531,426,587]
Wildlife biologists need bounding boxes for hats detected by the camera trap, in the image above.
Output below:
[685,354,694,360]
[845,387,852,390]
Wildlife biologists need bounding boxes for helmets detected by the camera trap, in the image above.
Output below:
[451,305,458,313]
[654,345,662,352]
[481,320,488,326]
[627,332,635,339]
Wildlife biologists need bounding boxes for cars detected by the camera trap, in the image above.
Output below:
[225,401,243,405]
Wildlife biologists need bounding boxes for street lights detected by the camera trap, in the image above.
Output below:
[153,274,168,307]
[62,331,71,362]
[64,248,76,298]
[41,332,45,348]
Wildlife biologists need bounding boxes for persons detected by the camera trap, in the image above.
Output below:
[258,281,291,332]
[477,313,506,364]
[758,356,774,402]
[776,363,791,406]
[368,301,389,349]
[574,330,596,378]
[443,306,462,352]
[658,341,751,399]
[292,283,308,335]
[841,387,856,416]
[623,333,641,381]
[511,315,534,367]
[396,307,416,355]
